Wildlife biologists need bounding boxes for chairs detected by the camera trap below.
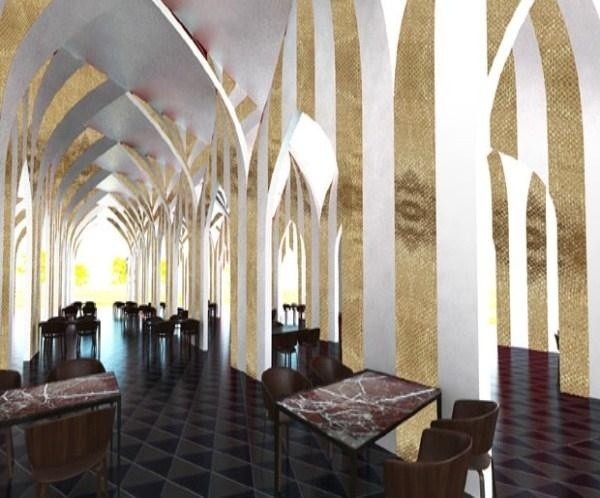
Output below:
[0,358,121,498]
[38,301,201,359]
[261,355,500,498]
[272,308,320,367]
